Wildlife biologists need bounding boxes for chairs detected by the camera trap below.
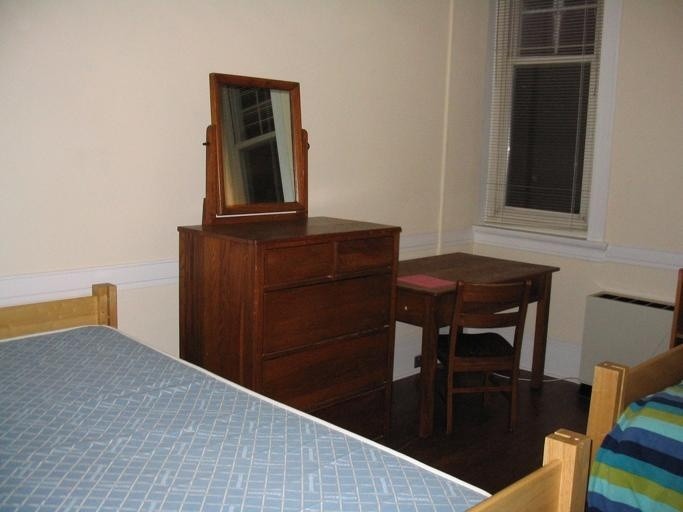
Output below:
[436,277,533,438]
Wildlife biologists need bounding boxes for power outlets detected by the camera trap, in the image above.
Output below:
[414,355,421,368]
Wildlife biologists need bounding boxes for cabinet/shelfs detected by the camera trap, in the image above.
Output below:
[177,218,402,446]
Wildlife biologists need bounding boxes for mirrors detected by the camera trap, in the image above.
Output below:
[201,73,311,229]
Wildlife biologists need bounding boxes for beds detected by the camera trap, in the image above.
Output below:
[0,285,593,512]
[584,342,683,512]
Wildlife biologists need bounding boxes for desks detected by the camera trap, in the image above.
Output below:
[396,251,561,440]
